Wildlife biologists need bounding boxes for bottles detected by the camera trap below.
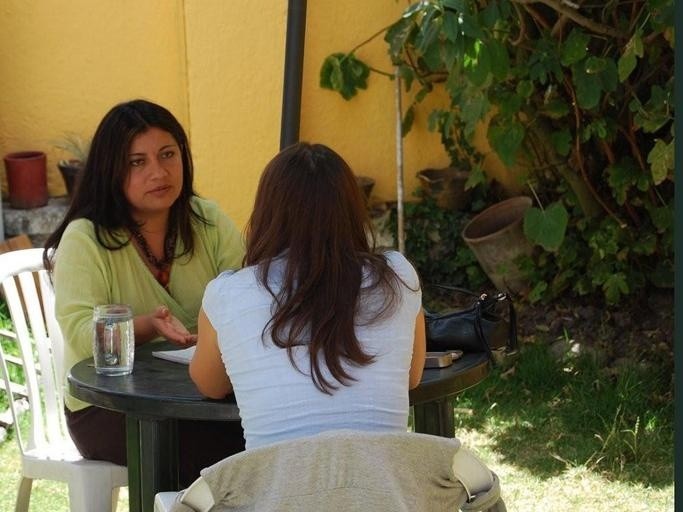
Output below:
[91,304,135,377]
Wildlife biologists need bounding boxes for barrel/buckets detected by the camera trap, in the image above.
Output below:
[462,194,544,293]
[4,150,49,210]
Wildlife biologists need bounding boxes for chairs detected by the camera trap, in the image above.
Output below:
[0,246,128,511]
[153,429,506,511]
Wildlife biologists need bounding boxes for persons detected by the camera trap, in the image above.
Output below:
[42,99,251,490]
[190,140,427,452]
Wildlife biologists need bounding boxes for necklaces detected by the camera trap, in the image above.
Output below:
[123,211,176,285]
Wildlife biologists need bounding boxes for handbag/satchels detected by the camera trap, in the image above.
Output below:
[422,293,519,369]
